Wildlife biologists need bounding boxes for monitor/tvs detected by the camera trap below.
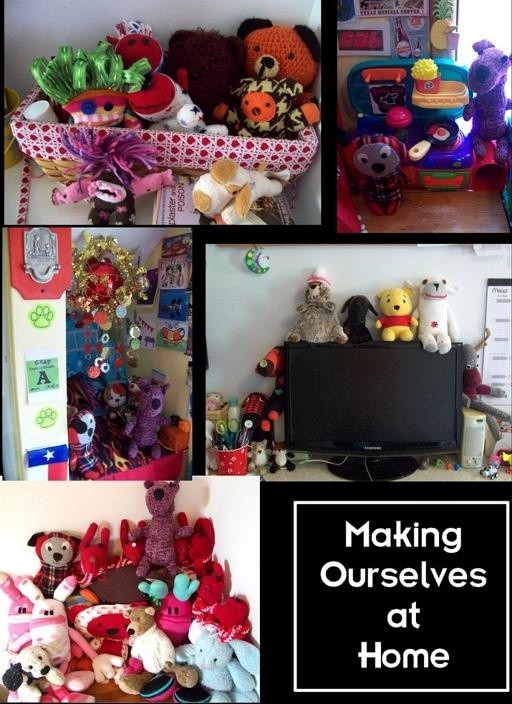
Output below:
[283,339,463,482]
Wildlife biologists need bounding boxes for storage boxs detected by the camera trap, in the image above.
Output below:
[70,412,183,480]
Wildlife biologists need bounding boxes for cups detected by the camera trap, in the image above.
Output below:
[213,438,248,476]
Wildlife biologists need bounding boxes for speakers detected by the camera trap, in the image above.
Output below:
[458,405,487,470]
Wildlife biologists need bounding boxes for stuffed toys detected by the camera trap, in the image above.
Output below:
[27,17,322,228]
[246,264,511,474]
[247,267,512,473]
[1,478,261,702]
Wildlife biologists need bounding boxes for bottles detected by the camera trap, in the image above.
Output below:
[394,16,411,57]
[413,35,422,57]
[228,398,239,430]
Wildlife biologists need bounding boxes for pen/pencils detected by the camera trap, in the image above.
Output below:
[212,418,252,451]
